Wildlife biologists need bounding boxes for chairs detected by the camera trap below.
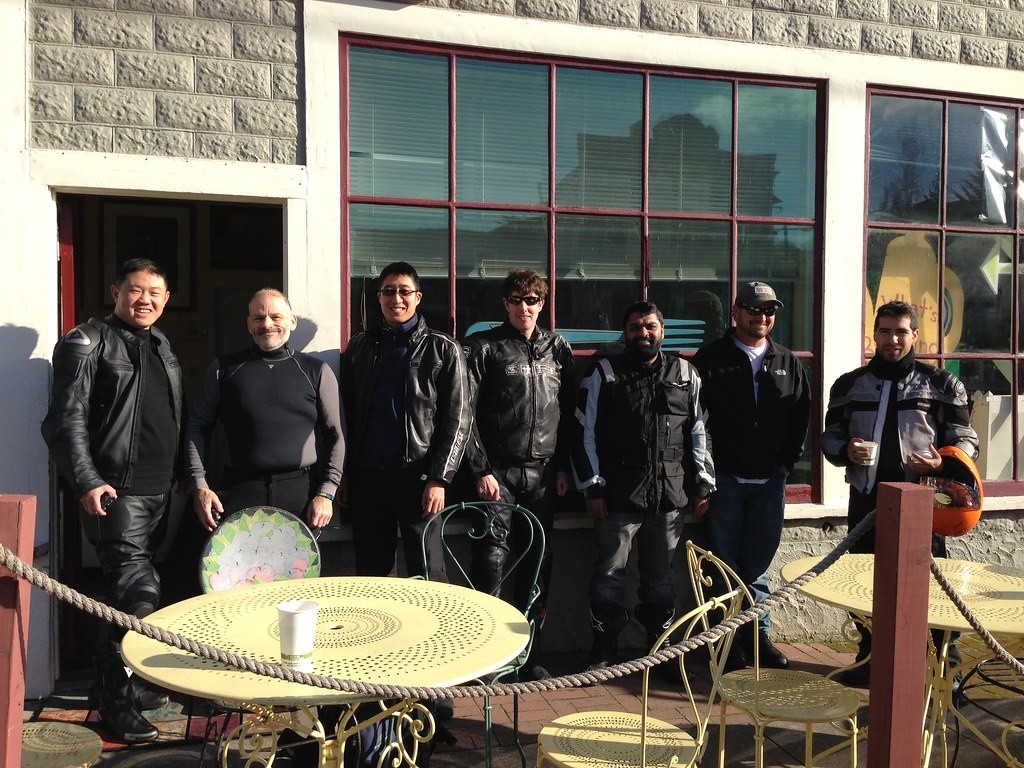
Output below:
[0,500,1024,768]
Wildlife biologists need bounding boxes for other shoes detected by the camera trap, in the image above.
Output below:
[131,686,169,710]
[943,664,969,708]
[531,663,554,681]
[841,661,870,686]
[98,698,160,744]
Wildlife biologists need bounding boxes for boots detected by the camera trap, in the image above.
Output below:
[705,595,746,671]
[740,621,789,669]
[572,600,631,687]
[635,603,696,685]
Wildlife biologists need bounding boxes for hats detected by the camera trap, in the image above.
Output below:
[736,281,785,311]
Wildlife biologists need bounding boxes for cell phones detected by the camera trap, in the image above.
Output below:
[100,492,115,511]
[211,508,221,525]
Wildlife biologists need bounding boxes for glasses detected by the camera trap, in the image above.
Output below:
[380,286,420,297]
[505,294,544,306]
[741,305,776,318]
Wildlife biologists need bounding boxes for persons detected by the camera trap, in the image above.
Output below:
[565,298,717,683]
[821,299,981,696]
[339,262,474,584]
[188,287,345,542]
[40,260,182,740]
[462,268,577,682]
[690,280,812,672]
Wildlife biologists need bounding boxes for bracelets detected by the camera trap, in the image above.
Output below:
[317,492,333,500]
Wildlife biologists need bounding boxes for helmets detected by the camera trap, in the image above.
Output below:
[920,447,984,537]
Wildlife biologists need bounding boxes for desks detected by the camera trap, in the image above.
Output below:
[120,577,531,768]
[779,550,1024,768]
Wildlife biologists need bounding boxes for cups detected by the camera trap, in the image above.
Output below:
[276,600,319,674]
[854,440,878,465]
